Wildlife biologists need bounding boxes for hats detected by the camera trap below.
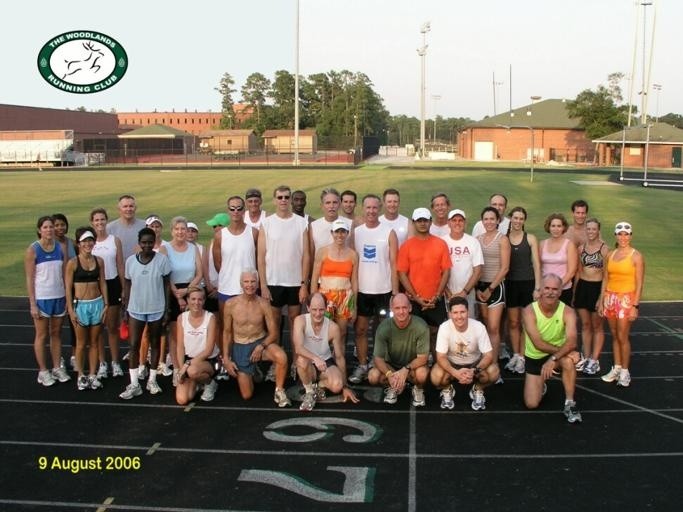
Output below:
[448,209,466,220]
[331,219,350,232]
[146,216,164,227]
[187,223,199,232]
[206,213,230,227]
[79,231,94,241]
[615,222,632,235]
[411,207,432,222]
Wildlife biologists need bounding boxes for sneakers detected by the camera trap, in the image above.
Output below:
[216,367,229,381]
[412,385,426,407]
[200,379,218,402]
[563,401,583,423]
[576,357,631,388]
[255,363,326,412]
[37,347,179,400]
[439,384,456,410]
[348,365,373,384]
[384,388,398,405]
[469,384,486,411]
[494,341,525,386]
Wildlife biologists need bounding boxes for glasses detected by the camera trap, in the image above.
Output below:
[277,195,290,200]
[229,206,243,211]
[617,224,631,229]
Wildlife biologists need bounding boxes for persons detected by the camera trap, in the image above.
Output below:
[597,220,645,392]
[64,183,611,426]
[50,214,77,374]
[24,215,71,387]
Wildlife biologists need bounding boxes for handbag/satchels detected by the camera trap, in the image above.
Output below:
[119,319,129,341]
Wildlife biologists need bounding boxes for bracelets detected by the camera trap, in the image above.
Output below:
[632,304,640,310]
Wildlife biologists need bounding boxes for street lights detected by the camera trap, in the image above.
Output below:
[651,82,661,123]
[414,20,431,160]
